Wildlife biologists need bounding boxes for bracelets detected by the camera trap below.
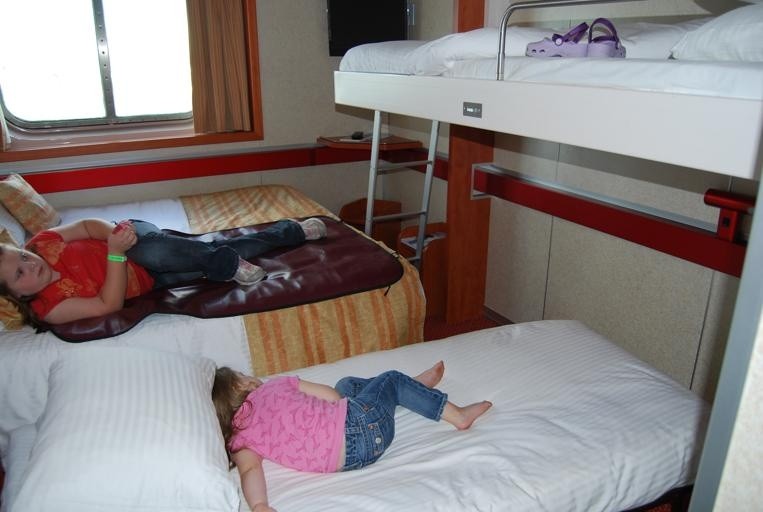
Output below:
[107,254,127,264]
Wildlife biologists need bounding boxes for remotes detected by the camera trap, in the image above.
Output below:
[352,132,363,139]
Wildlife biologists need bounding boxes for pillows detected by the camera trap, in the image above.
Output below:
[5,346,243,511]
[1,172,62,235]
[1,226,29,331]
[673,4,761,63]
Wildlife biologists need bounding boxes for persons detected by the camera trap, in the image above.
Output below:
[209,358,493,512]
[0,217,329,324]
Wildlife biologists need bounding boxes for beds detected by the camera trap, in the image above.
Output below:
[334,2,761,280]
[2,184,425,428]
[1,320,709,511]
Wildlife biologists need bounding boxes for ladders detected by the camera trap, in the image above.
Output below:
[341,198,401,248]
[366,111,440,269]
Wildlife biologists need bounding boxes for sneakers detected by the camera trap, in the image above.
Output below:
[224,255,265,286]
[279,218,328,241]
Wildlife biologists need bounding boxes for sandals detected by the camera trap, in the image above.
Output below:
[525,18,626,58]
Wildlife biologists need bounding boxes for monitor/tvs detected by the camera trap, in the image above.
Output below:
[327,0,408,57]
